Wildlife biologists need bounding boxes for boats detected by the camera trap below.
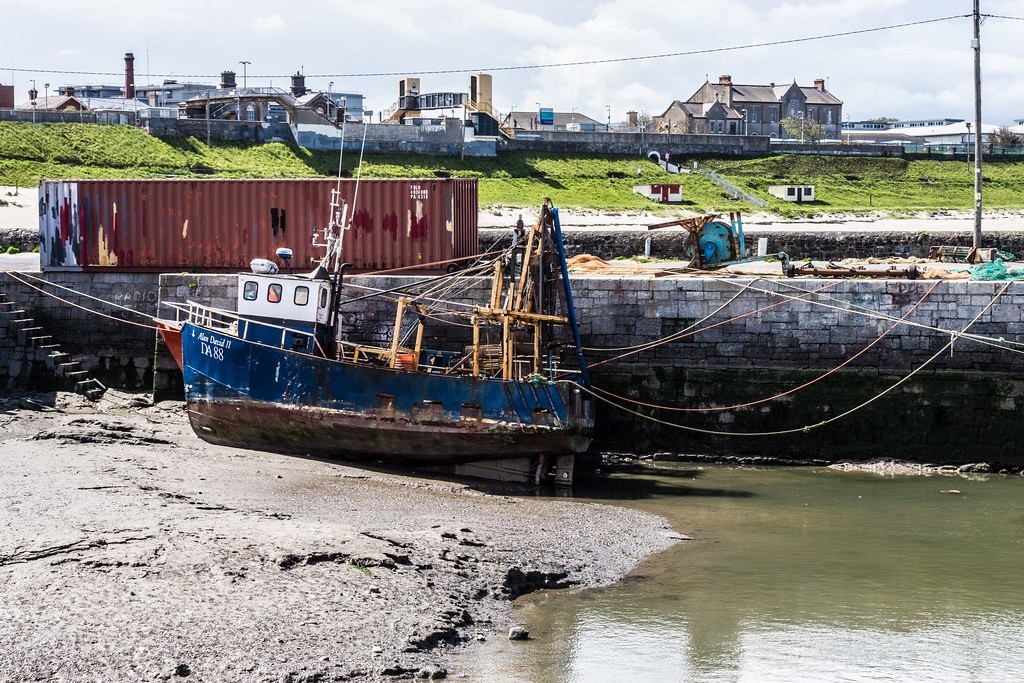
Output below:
[152,99,605,490]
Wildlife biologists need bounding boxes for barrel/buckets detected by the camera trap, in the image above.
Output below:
[393,353,417,370]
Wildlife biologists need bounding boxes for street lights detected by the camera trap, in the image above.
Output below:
[536,103,540,110]
[29,79,35,124]
[43,83,51,112]
[606,104,611,126]
[798,111,804,144]
[328,82,334,100]
[965,122,971,171]
[238,60,251,92]
[130,84,137,125]
[571,107,578,114]
[511,105,517,112]
[741,109,748,135]
